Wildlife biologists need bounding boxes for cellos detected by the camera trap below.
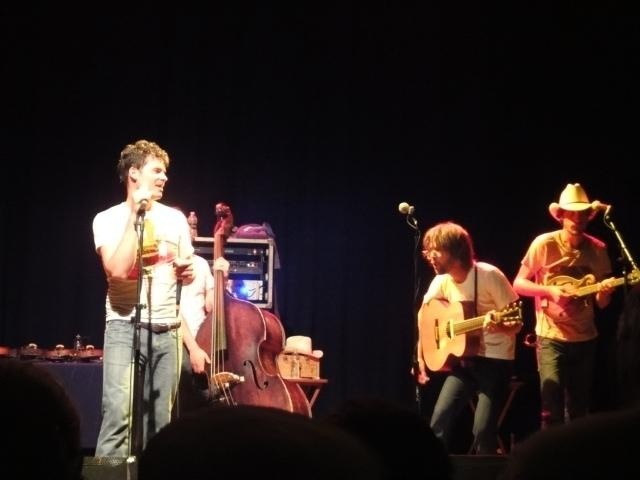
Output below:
[191,204,312,417]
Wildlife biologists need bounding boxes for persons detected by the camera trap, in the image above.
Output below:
[4,354,640,480]
[410,222,524,455]
[512,184,616,430]
[177,206,230,411]
[92,139,196,458]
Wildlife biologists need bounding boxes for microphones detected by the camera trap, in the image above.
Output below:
[137,200,148,217]
[175,267,186,317]
[591,199,614,210]
[398,202,424,230]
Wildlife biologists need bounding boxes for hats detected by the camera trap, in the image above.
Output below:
[283,334,324,359]
[548,183,600,223]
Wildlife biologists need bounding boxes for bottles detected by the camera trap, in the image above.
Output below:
[73,333,82,362]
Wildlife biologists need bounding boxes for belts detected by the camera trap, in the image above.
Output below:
[130,320,182,334]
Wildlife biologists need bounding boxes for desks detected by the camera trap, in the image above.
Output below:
[1,359,104,455]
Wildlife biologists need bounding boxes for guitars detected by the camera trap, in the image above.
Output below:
[418,297,526,372]
